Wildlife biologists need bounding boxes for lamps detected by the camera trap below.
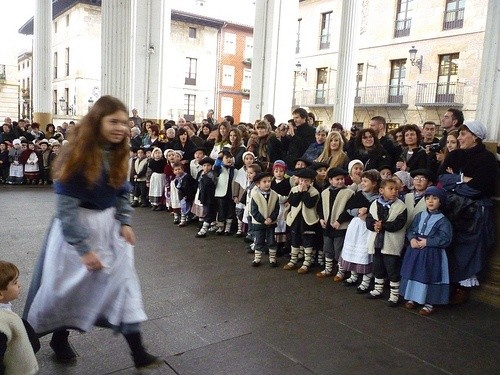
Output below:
[409,46,423,74]
[296,61,307,81]
[59,97,73,115]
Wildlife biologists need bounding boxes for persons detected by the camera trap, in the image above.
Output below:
[0,260,39,375]
[127,108,500,315]
[24,95,166,375]
[0,117,76,184]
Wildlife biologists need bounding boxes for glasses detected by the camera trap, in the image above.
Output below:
[413,177,426,182]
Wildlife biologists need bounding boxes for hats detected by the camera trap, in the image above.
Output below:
[327,167,348,177]
[13,137,69,147]
[410,168,432,181]
[424,186,446,204]
[295,169,318,178]
[199,157,215,167]
[313,162,330,171]
[151,148,162,157]
[253,172,272,183]
[222,147,233,157]
[348,160,363,175]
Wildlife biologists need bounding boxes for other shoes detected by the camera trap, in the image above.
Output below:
[131,200,435,316]
[7,179,48,185]
[449,288,468,304]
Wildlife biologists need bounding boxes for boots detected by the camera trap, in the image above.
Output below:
[50,329,75,359]
[130,346,164,373]
